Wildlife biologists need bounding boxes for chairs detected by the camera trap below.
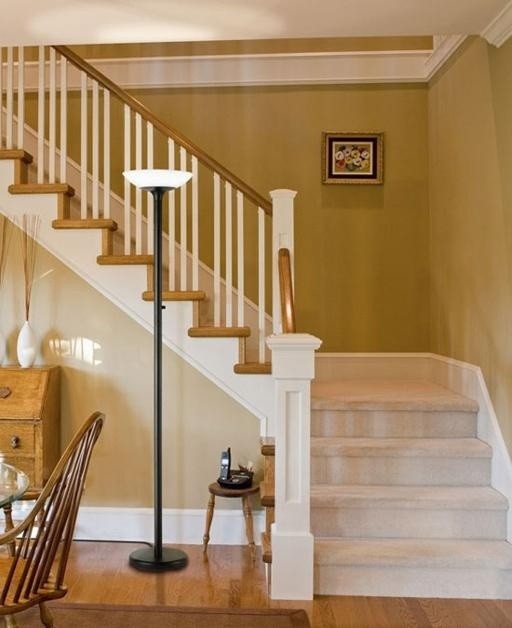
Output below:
[1,409,106,628]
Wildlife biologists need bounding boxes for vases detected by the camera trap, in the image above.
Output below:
[16,320,38,369]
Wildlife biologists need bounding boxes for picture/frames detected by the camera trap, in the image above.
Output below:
[320,129,384,186]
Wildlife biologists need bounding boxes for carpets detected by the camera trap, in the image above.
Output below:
[2,601,311,628]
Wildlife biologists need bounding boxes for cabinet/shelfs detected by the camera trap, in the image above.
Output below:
[0,364,62,500]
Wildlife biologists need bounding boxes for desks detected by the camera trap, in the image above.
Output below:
[202,483,260,570]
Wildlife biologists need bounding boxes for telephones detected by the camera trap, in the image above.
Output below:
[217,448,254,488]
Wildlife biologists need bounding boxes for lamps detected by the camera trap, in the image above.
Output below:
[122,168,194,575]
